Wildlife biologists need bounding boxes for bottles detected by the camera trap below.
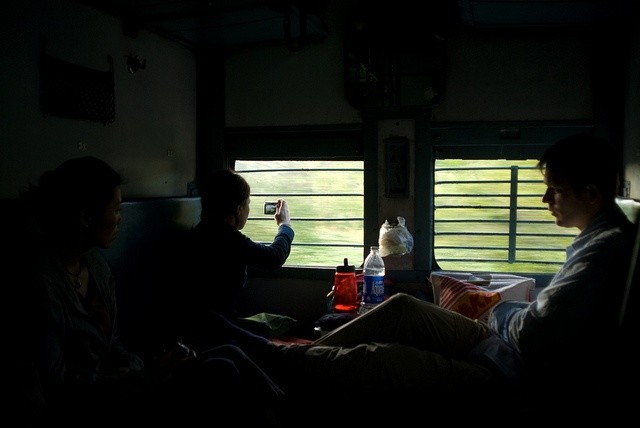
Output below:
[362,242,386,304]
[333,257,359,314]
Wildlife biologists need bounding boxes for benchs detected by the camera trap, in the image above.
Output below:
[611,194,640,327]
[109,197,202,273]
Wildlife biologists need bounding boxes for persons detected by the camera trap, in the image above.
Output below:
[284,134,640,426]
[0,156,284,427]
[138,168,293,329]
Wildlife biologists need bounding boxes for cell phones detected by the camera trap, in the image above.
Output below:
[264,203,277,215]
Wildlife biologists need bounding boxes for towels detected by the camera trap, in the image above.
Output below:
[439,275,501,320]
